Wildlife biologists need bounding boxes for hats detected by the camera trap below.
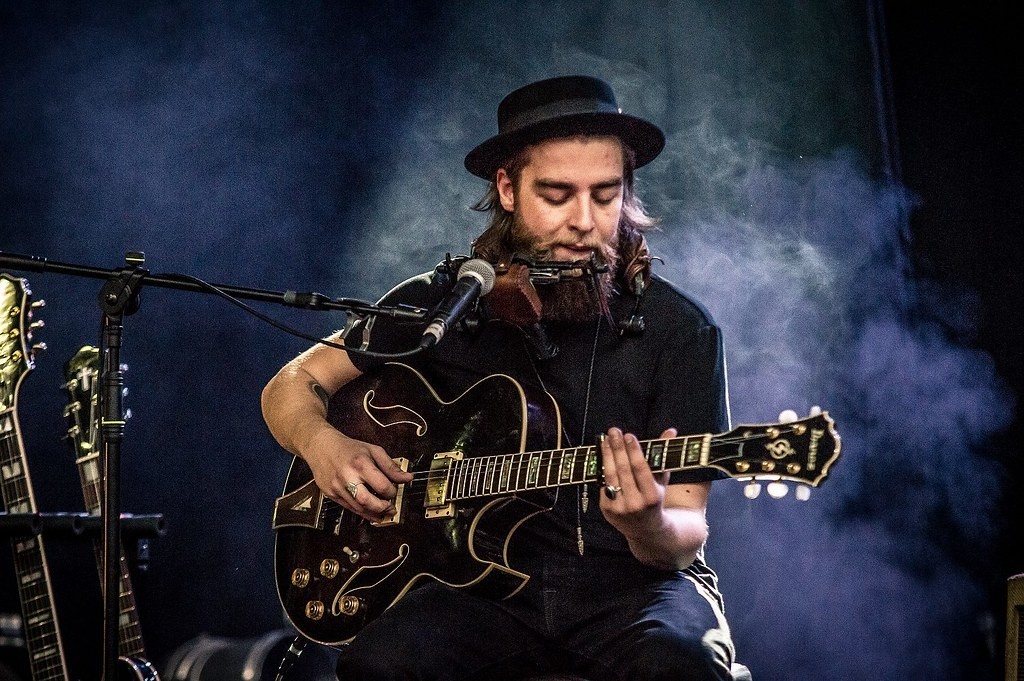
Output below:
[465,74,666,184]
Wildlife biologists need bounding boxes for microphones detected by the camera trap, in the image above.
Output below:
[421,259,498,351]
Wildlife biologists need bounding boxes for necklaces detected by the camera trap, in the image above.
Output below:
[517,310,605,559]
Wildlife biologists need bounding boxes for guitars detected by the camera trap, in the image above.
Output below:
[0,272,70,681]
[273,363,843,646]
[59,346,156,681]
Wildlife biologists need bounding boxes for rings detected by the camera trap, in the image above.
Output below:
[603,483,622,502]
[345,481,363,500]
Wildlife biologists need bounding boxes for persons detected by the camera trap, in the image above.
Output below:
[259,72,740,681]
